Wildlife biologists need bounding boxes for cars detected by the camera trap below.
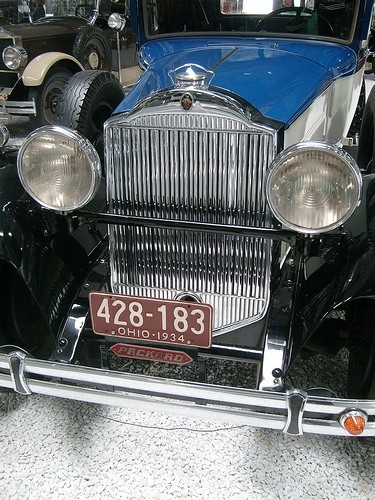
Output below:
[0,0,375,440]
[0,0,219,96]
